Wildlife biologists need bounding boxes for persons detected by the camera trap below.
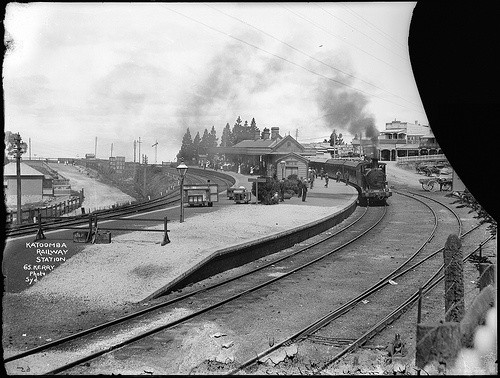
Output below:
[315,167,318,179]
[312,167,315,178]
[345,170,349,186]
[310,173,314,189]
[320,167,325,180]
[198,157,255,175]
[336,169,342,183]
[302,177,307,202]
[308,166,312,178]
[297,177,303,197]
[279,177,286,203]
[323,172,329,187]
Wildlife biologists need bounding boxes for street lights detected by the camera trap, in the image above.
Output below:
[175,156,188,222]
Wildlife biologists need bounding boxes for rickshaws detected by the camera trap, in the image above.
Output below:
[419,176,440,192]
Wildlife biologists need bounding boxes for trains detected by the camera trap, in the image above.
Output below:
[308,155,392,205]
